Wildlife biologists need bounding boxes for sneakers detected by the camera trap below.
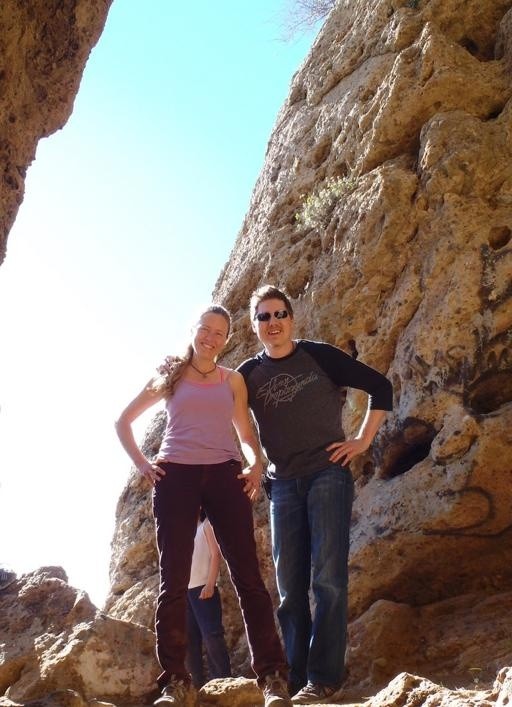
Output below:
[153,680,192,707]
[260,671,295,707]
[287,677,347,705]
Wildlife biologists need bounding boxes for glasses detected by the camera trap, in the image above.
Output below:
[254,310,290,321]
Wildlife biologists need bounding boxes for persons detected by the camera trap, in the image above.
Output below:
[114,304,295,707]
[235,283,395,705]
[189,505,232,691]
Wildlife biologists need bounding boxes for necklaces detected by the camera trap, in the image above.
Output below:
[187,358,218,378]
